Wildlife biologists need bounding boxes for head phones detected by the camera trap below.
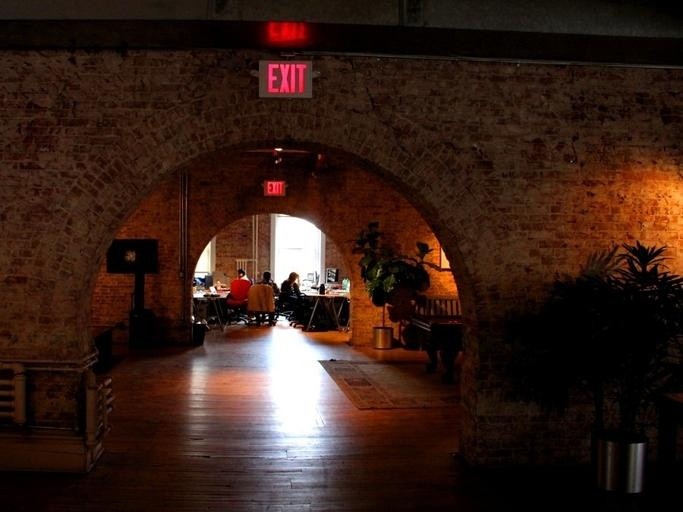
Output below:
[240,268,243,276]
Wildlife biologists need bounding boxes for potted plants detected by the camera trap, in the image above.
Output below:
[541,242,683,494]
[346,222,433,349]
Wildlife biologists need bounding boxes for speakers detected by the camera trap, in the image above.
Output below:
[107,238,158,273]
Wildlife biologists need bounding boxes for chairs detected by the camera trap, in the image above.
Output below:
[227,279,315,328]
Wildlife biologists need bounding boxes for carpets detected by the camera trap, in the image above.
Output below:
[318,356,470,410]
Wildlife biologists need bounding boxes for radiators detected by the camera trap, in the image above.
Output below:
[235,259,256,280]
[0,362,26,427]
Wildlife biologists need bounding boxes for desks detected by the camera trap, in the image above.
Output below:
[192,289,230,332]
[303,292,345,331]
[406,314,464,385]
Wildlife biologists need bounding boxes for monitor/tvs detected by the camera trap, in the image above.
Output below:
[326,268,339,283]
[205,275,213,290]
[311,271,319,288]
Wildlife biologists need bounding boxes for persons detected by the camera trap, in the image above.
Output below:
[281,271,304,323]
[254,270,280,327]
[238,268,250,281]
